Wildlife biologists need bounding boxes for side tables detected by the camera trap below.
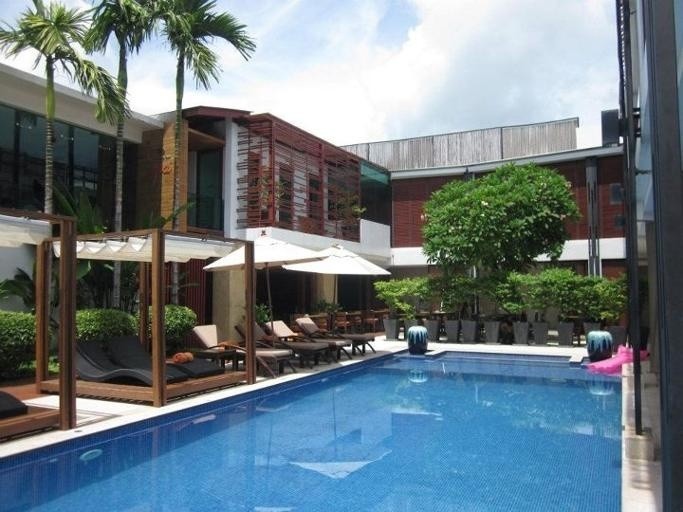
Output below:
[196,348,236,371]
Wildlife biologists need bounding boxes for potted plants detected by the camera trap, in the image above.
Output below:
[369,268,653,353]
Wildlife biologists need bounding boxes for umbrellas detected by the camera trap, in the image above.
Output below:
[282,242,393,307]
[200,231,332,348]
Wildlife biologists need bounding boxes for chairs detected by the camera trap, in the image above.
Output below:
[234,321,329,369]
[75,341,187,386]
[104,335,226,378]
[231,313,233,315]
[266,320,353,362]
[296,316,377,357]
[194,323,297,379]
[0,385,61,443]
